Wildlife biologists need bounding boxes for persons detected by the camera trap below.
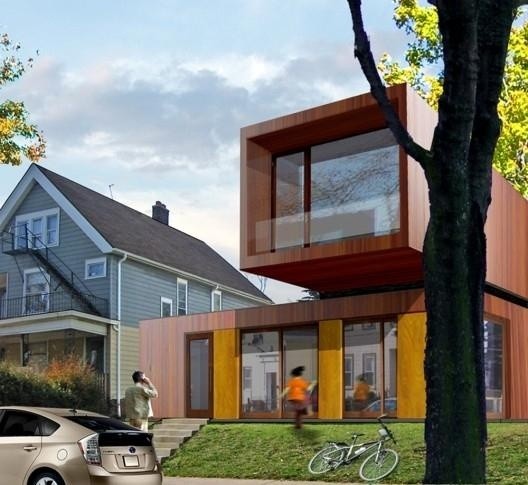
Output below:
[278,365,319,429]
[124,371,159,433]
[351,377,370,402]
